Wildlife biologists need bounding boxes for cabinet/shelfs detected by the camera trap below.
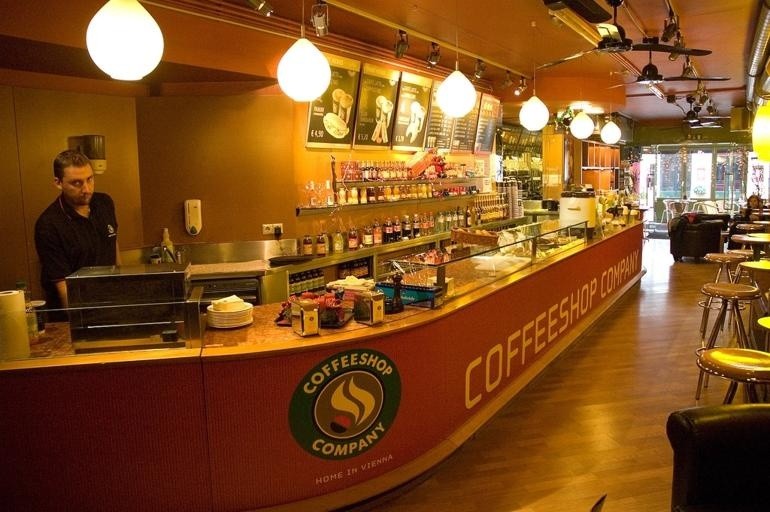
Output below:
[573,140,621,188]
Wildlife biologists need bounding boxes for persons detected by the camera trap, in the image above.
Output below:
[33,148,118,321]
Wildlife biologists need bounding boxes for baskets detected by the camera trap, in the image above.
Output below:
[450,226,499,246]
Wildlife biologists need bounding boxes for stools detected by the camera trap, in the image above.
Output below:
[737,224,763,249]
[700,253,746,333]
[694,348,770,405]
[728,250,754,258]
[700,282,760,351]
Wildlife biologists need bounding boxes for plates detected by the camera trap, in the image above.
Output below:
[206,301,253,329]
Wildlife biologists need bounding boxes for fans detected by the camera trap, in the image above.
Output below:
[535,0,712,71]
[663,55,732,81]
[604,49,731,88]
[688,106,723,130]
[682,96,732,123]
[544,0,613,23]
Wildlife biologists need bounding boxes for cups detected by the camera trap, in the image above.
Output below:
[492,175,525,219]
[26,300,46,335]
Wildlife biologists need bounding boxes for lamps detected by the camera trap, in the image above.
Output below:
[519,26,550,131]
[601,102,621,144]
[569,110,595,141]
[661,11,679,41]
[309,1,329,37]
[513,77,528,95]
[393,29,409,59]
[86,0,164,81]
[246,1,275,17]
[666,94,678,104]
[548,1,642,78]
[669,32,686,62]
[437,29,477,118]
[276,0,332,102]
[424,42,442,69]
[499,71,514,90]
[471,59,488,83]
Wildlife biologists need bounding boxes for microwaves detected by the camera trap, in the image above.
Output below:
[64,262,192,351]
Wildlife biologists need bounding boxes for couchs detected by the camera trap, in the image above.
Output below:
[666,403,770,512]
[687,212,730,242]
[667,217,723,262]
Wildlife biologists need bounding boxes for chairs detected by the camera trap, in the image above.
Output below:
[661,199,671,221]
[716,199,740,214]
[701,201,718,214]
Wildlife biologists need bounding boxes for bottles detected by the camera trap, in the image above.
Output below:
[336,183,433,205]
[333,231,343,253]
[348,228,358,251]
[323,234,329,254]
[149,247,161,264]
[316,235,326,256]
[289,269,325,296]
[15,282,38,346]
[356,160,407,180]
[382,205,464,244]
[361,223,382,248]
[321,179,335,206]
[304,181,321,207]
[161,228,173,263]
[466,205,471,227]
[460,164,467,178]
[339,258,369,279]
[303,235,313,257]
